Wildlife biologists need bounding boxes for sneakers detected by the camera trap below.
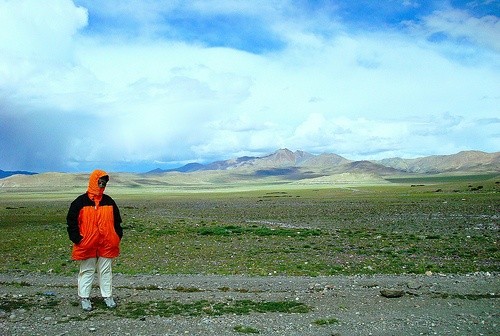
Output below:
[81,298,93,311]
[103,297,117,308]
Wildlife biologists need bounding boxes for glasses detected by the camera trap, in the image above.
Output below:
[98,181,106,186]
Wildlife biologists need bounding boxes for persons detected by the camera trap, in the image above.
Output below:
[66,169,123,311]
